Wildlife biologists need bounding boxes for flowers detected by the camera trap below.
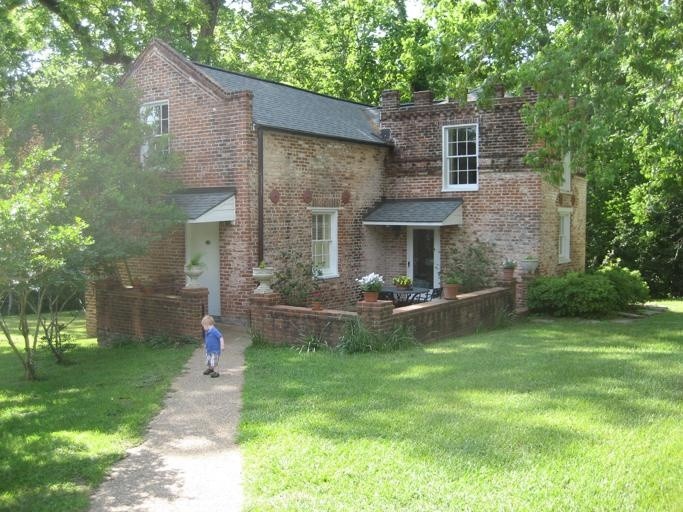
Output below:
[393,277,411,286]
[354,273,384,292]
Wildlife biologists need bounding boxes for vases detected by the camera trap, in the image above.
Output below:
[361,290,379,302]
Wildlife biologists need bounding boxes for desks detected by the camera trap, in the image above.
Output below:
[382,287,429,309]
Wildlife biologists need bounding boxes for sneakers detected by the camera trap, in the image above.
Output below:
[203,369,220,377]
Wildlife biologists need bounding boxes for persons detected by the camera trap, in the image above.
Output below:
[201,315,225,378]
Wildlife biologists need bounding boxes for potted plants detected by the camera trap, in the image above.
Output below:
[310,292,323,311]
[500,259,518,281]
[180,253,205,289]
[252,261,277,295]
[441,278,458,300]
[520,258,538,273]
